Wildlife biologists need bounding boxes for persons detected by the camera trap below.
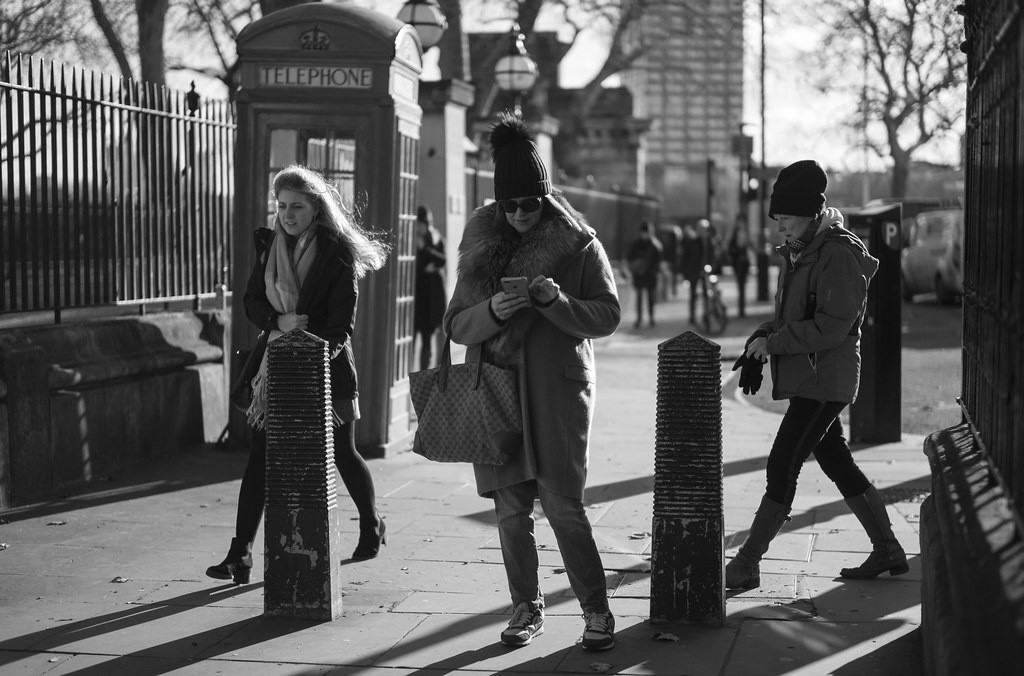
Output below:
[415,207,446,371]
[628,221,664,328]
[658,213,754,325]
[441,117,622,651]
[725,159,908,589]
[206,166,387,584]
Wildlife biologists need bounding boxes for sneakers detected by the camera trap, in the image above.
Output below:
[500,593,546,646]
[581,610,615,651]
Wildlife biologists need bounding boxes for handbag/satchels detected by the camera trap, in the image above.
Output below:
[408,307,524,467]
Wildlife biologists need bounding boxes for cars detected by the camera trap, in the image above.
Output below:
[898,208,966,305]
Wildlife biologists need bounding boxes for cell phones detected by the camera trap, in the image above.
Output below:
[501,276,532,308]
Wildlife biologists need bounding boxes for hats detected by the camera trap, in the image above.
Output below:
[417,204,430,226]
[640,218,652,233]
[488,109,552,201]
[768,159,828,221]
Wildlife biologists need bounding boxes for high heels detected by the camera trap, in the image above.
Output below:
[205,537,254,584]
[351,509,388,561]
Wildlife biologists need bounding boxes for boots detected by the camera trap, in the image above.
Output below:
[690,295,696,323]
[724,495,793,591]
[419,348,431,370]
[647,302,655,327]
[634,303,642,328]
[839,483,911,581]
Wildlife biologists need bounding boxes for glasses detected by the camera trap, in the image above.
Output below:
[502,197,541,213]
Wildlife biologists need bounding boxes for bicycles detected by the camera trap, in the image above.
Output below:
[691,272,731,337]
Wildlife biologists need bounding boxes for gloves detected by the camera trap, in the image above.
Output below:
[744,328,767,350]
[731,348,769,395]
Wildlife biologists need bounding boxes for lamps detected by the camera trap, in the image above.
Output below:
[494,21,540,119]
[396,0,449,53]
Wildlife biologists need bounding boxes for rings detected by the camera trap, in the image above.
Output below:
[504,308,509,313]
